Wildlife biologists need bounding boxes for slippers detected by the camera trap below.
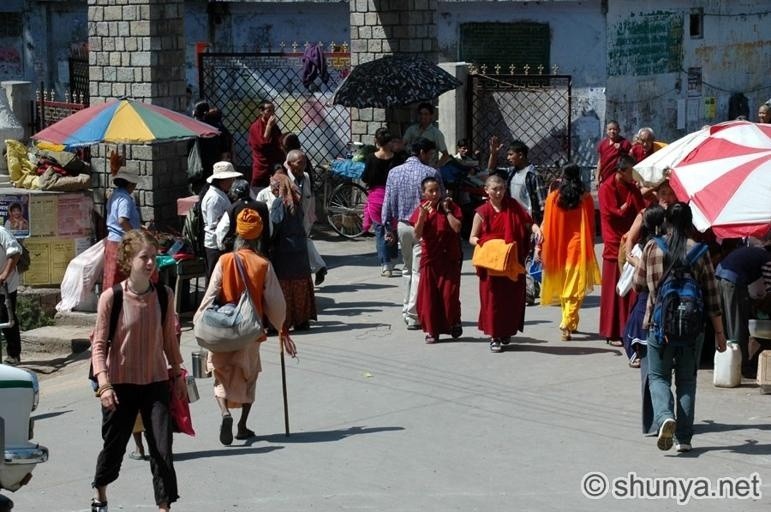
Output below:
[234,430,253,440]
[128,451,144,460]
[219,416,232,445]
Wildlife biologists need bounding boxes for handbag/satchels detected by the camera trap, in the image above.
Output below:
[189,144,203,181]
[193,289,263,354]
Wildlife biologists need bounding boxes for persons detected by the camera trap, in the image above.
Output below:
[5,201,30,231]
[362,102,771,453]
[0,226,21,367]
[88,100,328,511]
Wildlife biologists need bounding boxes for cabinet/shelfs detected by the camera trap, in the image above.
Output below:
[155,255,207,322]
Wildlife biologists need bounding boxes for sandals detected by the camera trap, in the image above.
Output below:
[489,339,500,353]
[450,324,462,339]
[425,334,438,344]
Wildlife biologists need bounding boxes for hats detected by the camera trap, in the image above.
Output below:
[206,161,242,183]
[112,166,137,183]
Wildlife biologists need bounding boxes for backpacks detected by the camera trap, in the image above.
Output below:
[16,240,29,272]
[653,236,708,348]
[181,183,208,253]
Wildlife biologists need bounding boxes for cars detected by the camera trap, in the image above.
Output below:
[1,352,50,508]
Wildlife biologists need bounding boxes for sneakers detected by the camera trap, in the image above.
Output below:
[657,418,676,450]
[676,444,691,452]
[315,266,326,285]
[2,356,19,367]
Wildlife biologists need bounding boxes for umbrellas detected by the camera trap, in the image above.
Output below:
[331,54,464,138]
[27,100,222,169]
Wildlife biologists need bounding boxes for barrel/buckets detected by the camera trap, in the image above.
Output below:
[712,337,744,388]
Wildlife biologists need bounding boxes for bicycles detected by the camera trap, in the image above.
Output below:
[310,159,373,239]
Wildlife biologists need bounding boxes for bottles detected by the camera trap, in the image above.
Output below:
[166,238,185,256]
